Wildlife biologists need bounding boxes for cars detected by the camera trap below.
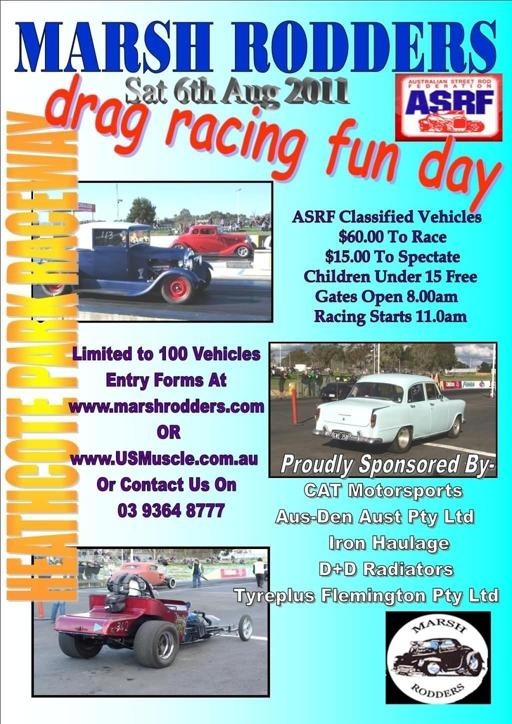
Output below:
[312,373,466,454]
[391,638,485,676]
[33,221,254,305]
[53,561,253,668]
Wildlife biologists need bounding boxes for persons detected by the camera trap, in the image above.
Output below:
[278,369,336,397]
[50,562,67,624]
[252,557,266,588]
[192,558,203,588]
[151,213,271,235]
